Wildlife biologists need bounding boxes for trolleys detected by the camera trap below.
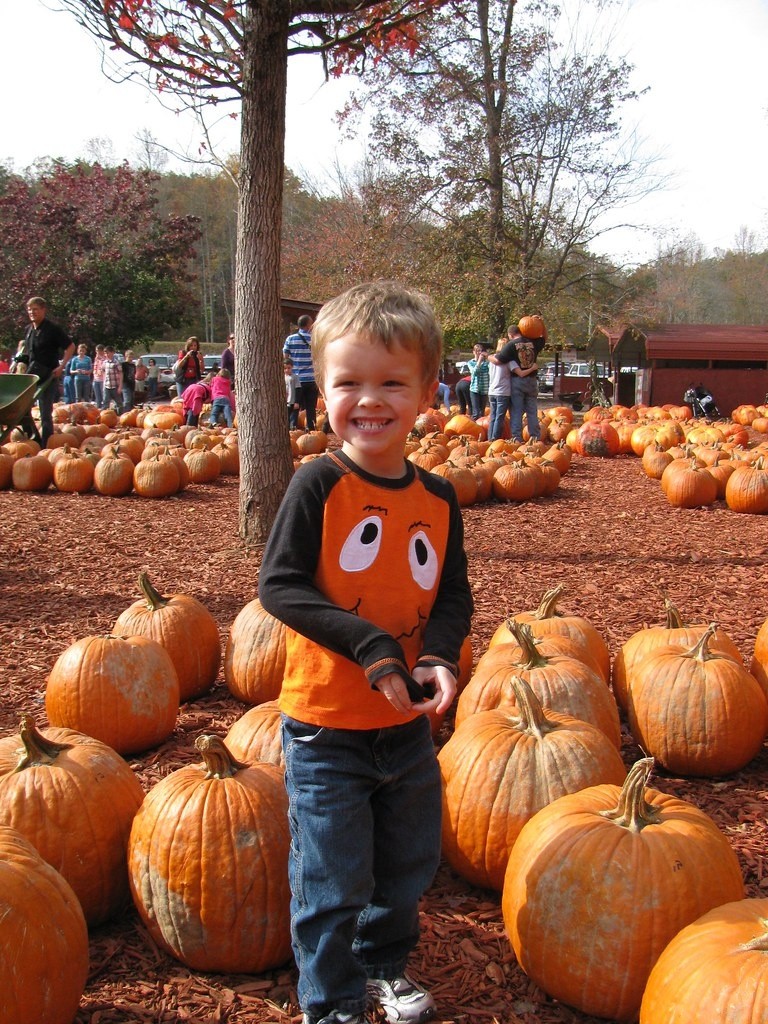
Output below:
[0,367,56,449]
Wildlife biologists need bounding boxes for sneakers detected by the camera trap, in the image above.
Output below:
[364,972,436,1024]
[300,1006,359,1024]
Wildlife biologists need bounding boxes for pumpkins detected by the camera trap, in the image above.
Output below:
[0,397,768,515]
[0,570,768,1024]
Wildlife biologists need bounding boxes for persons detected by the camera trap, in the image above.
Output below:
[172,332,236,428]
[487,315,548,444]
[10,297,75,450]
[283,315,319,431]
[438,383,455,413]
[0,340,26,373]
[284,358,302,431]
[257,281,475,1024]
[455,376,472,415]
[64,344,160,417]
[468,344,489,421]
[487,338,538,442]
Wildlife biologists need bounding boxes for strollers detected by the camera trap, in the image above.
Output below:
[687,382,722,422]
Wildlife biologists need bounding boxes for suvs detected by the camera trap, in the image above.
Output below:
[201,354,223,380]
[542,362,570,390]
[139,355,177,383]
[564,363,607,379]
